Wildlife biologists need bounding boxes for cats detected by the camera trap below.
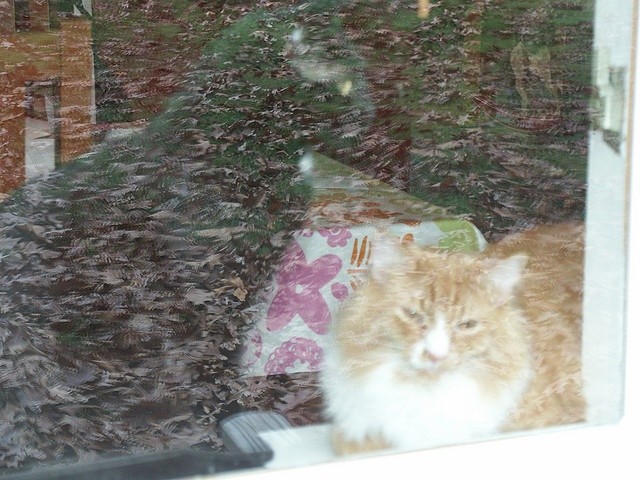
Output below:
[325,223,585,459]
